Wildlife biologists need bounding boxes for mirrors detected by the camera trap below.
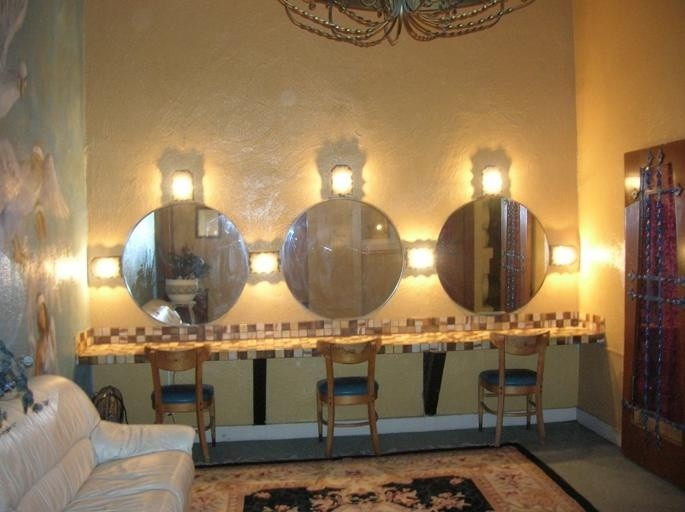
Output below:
[123,203,251,326]
[283,199,405,321]
[437,197,550,315]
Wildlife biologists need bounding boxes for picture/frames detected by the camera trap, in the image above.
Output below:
[195,207,222,238]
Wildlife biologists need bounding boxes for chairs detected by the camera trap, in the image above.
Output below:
[143,346,216,462]
[172,301,197,323]
[317,337,383,458]
[477,333,548,446]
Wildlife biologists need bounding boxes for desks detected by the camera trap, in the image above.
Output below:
[78,326,605,426]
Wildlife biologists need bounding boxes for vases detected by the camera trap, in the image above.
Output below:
[165,279,198,300]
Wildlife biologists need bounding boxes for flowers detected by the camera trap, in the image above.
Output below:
[158,243,210,279]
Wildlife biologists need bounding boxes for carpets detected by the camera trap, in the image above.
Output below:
[191,442,599,512]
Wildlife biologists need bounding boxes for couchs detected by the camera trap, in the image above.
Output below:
[141,299,183,325]
[0,375,195,511]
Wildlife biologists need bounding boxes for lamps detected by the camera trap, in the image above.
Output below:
[171,169,194,203]
[279,0,537,48]
[251,251,279,273]
[332,164,354,195]
[550,245,575,265]
[91,257,120,280]
[483,167,503,195]
[625,174,641,198]
[407,247,434,268]
